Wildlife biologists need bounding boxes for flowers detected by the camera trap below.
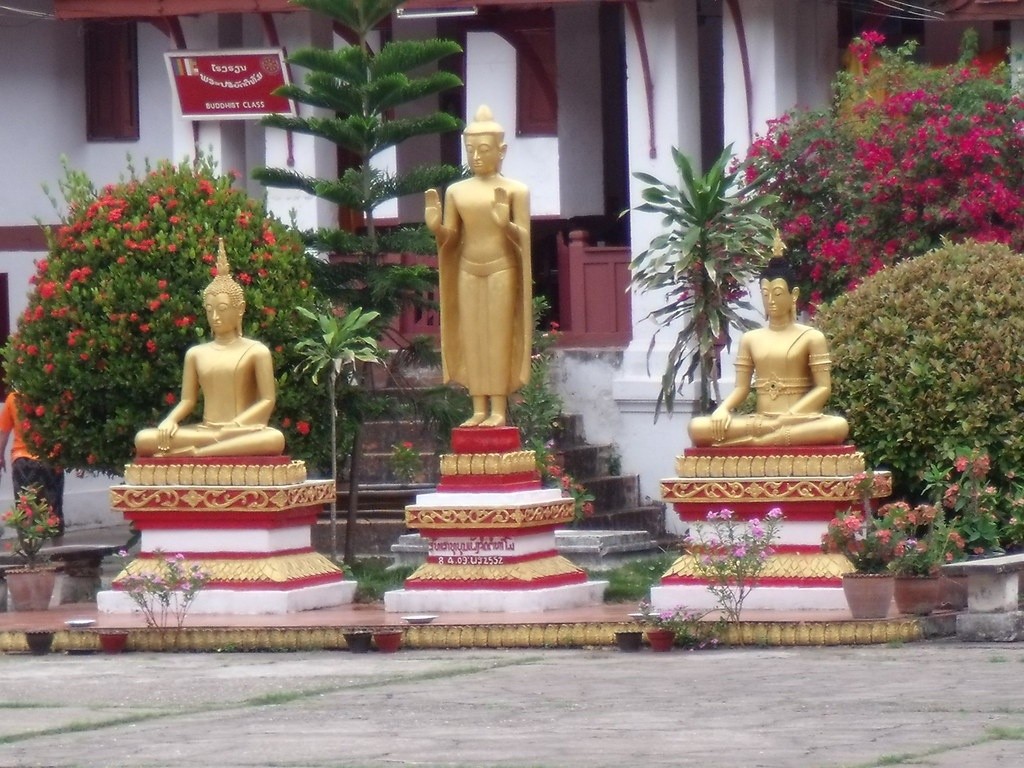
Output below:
[820,448,1024,579]
[637,600,694,633]
[1,486,61,569]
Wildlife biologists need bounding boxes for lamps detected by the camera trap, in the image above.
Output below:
[396,4,478,19]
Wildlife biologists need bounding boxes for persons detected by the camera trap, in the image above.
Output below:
[134,237,284,458]
[689,229,849,447]
[0,392,64,549]
[424,104,531,428]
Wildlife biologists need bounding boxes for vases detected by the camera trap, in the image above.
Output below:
[343,631,373,654]
[840,573,897,620]
[890,571,942,615]
[98,631,129,655]
[615,620,643,653]
[25,631,56,656]
[373,629,404,653]
[4,566,56,611]
[646,628,675,652]
